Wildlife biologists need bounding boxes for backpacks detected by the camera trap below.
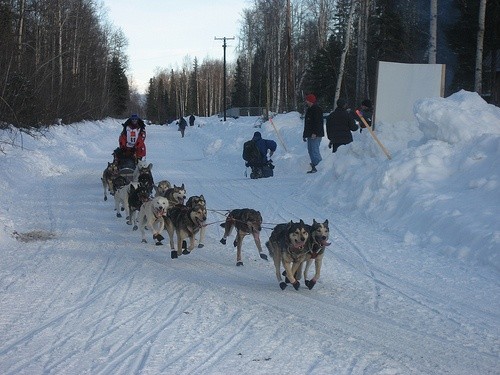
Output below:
[242,140,260,167]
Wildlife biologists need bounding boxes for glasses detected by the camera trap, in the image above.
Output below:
[131,118,137,120]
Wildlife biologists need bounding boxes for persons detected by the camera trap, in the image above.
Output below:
[189,112,195,126]
[350,99,372,133]
[303,94,324,174]
[118,113,146,169]
[243,132,277,179]
[178,117,187,138]
[326,99,360,153]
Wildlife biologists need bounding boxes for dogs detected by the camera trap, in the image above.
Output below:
[219,208,269,267]
[263,218,332,291]
[100,158,208,260]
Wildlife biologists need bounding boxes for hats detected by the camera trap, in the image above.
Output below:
[337,98,345,106]
[354,106,361,111]
[306,94,317,103]
[254,132,261,137]
[181,116,183,118]
[131,113,138,118]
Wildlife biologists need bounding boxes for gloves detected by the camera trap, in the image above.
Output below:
[311,134,316,141]
